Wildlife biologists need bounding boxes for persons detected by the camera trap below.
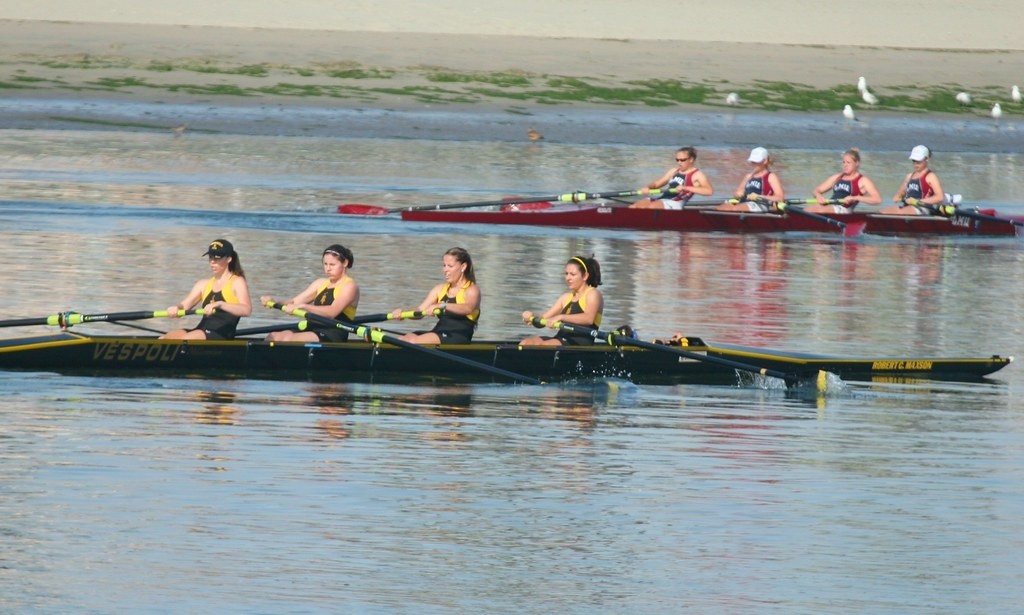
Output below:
[392,247,481,344]
[802,146,881,213]
[628,144,713,209]
[261,244,360,341]
[519,253,604,345]
[715,147,784,212]
[879,145,944,214]
[156,239,252,340]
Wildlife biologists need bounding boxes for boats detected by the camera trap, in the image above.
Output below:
[0,328,1015,381]
[400,198,1024,237]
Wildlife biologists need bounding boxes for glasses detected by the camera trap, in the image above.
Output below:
[676,156,691,162]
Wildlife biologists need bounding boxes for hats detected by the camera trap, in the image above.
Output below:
[202,240,234,257]
[909,145,929,161]
[747,147,769,163]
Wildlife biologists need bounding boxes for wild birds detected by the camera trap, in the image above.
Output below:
[1010,85,1023,102]
[990,103,1003,122]
[956,93,973,107]
[725,92,740,107]
[857,77,880,105]
[527,128,544,144]
[843,104,857,119]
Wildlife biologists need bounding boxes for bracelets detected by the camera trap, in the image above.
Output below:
[441,300,446,313]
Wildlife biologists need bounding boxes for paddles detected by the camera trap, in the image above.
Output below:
[750,194,882,236]
[233,310,448,337]
[0,307,217,328]
[335,186,676,215]
[520,311,843,393]
[681,198,854,205]
[265,298,640,396]
[900,196,1023,230]
[501,193,764,219]
